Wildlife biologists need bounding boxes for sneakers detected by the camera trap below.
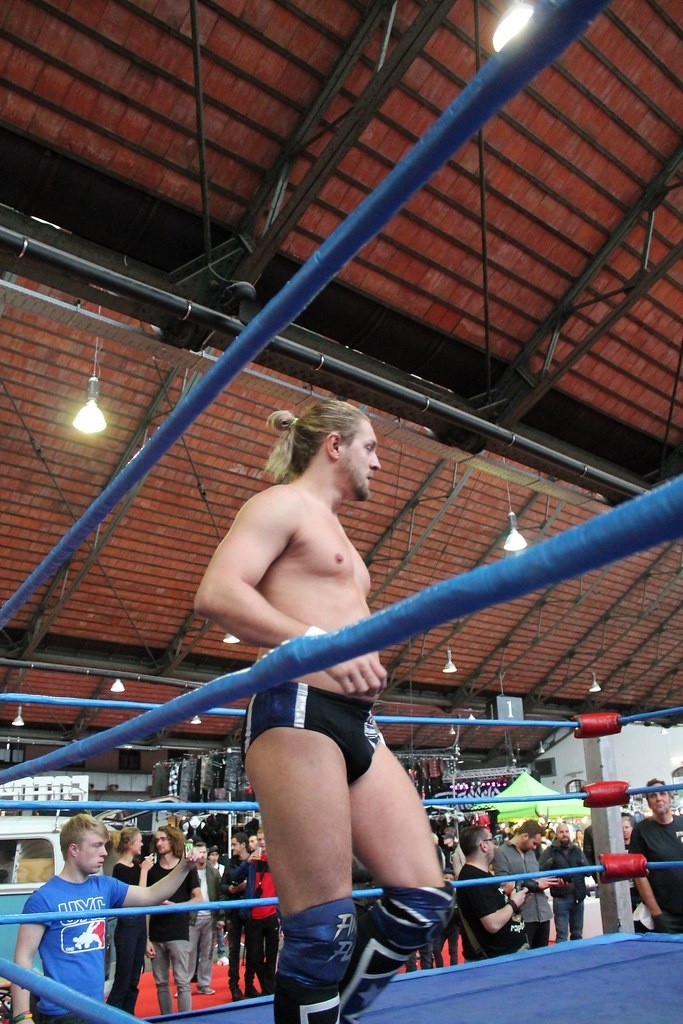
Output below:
[196,987,215,995]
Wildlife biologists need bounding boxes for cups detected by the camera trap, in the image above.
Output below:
[256,846,263,860]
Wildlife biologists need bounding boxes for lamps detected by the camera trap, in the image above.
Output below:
[72,303,106,433]
[111,679,125,692]
[492,0,533,51]
[224,631,239,645]
[469,713,476,720]
[191,715,203,725]
[443,640,458,672]
[589,673,601,692]
[13,697,25,726]
[538,740,551,754]
[447,717,457,736]
[501,475,526,553]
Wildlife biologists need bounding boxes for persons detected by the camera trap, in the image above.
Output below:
[189,827,279,1001]
[9,814,204,1024]
[192,399,458,1024]
[429,820,559,963]
[538,824,594,942]
[107,826,157,1015]
[143,825,203,1015]
[582,778,683,934]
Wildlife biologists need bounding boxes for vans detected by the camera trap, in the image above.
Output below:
[0,816,104,989]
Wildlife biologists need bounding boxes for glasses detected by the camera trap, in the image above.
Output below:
[479,838,497,846]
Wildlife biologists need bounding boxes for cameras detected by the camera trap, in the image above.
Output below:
[520,879,538,895]
[552,875,574,883]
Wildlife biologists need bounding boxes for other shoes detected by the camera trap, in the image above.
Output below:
[245,989,262,998]
[233,993,242,1001]
[217,956,229,966]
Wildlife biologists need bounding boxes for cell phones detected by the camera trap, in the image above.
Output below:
[184,843,193,858]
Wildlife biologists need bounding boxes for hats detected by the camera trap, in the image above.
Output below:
[207,847,221,860]
[441,833,455,839]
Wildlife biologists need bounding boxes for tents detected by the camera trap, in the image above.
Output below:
[470,772,590,824]
